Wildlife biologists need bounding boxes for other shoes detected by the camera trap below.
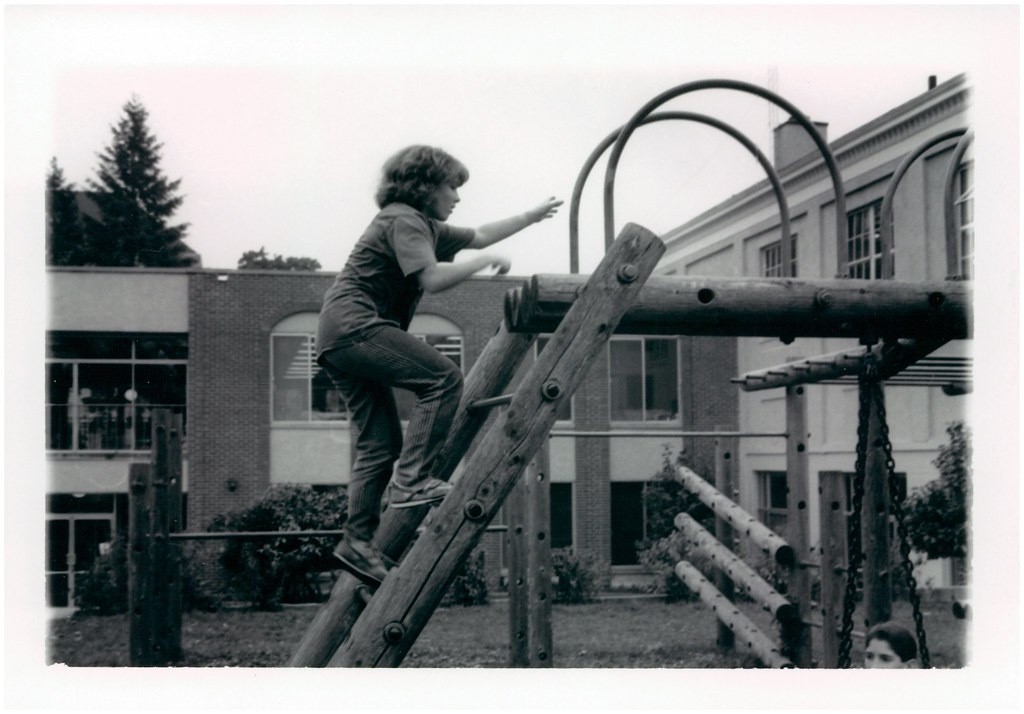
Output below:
[390,479,452,509]
[332,540,389,583]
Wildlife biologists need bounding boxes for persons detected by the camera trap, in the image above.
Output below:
[315,144,564,588]
[864,622,917,668]
